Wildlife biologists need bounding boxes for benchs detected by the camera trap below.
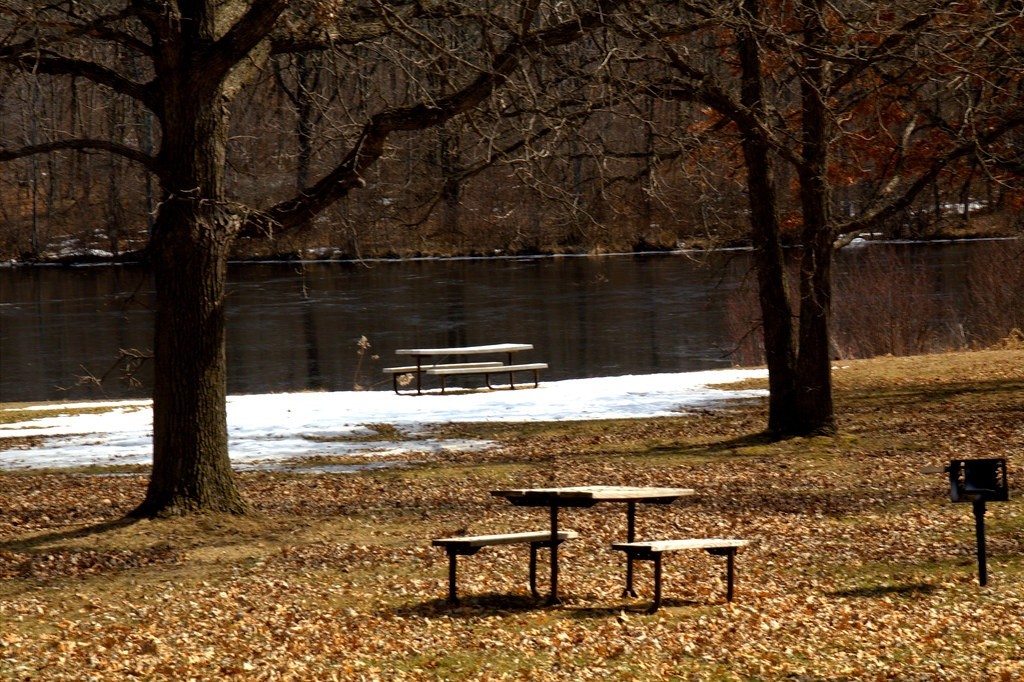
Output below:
[382,360,503,390]
[433,531,578,599]
[610,540,746,600]
[426,362,548,389]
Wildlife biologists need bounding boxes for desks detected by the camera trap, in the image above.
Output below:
[393,343,534,393]
[489,486,694,600]
